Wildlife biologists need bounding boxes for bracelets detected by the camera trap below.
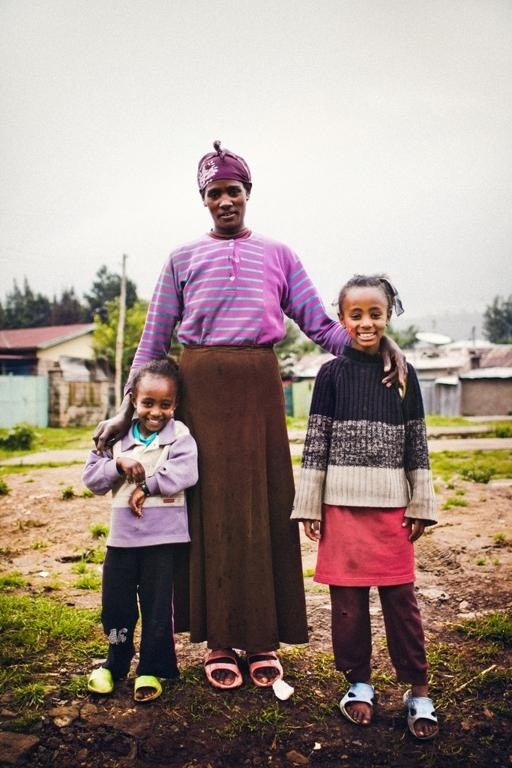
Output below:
[140,483,151,497]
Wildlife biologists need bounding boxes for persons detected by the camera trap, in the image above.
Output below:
[88,139,409,692]
[286,273,441,743]
[84,358,203,705]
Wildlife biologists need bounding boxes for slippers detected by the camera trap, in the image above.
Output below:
[88,667,114,694]
[245,650,284,687]
[134,674,162,702]
[205,650,243,690]
[403,688,440,740]
[340,682,375,726]
